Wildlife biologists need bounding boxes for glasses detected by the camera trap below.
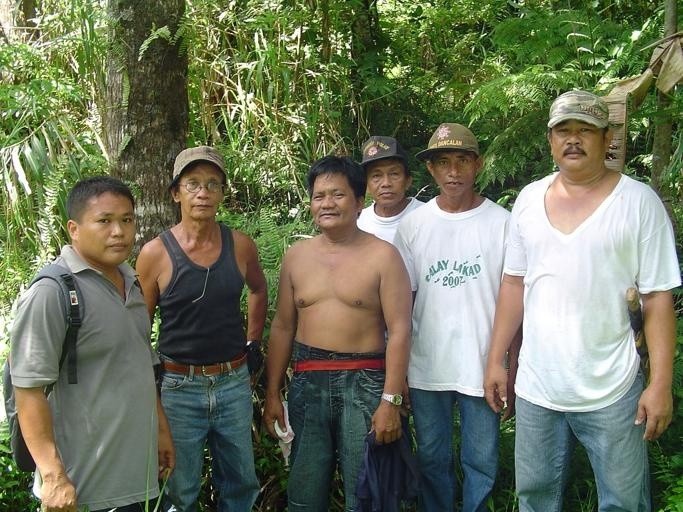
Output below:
[177,181,226,193]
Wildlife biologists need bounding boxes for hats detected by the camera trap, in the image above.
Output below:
[173,146,229,183]
[547,90,609,129]
[359,136,409,166]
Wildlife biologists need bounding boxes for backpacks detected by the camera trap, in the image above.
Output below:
[1,264,145,472]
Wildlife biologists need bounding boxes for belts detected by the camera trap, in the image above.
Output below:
[162,353,247,375]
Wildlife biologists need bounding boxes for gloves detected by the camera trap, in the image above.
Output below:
[245,339,263,375]
[152,363,164,398]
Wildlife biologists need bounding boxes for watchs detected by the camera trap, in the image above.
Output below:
[246,341,264,375]
[380,393,403,406]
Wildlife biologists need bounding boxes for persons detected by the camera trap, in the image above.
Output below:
[355,136,427,512]
[133,146,268,512]
[483,90,682,512]
[392,122,522,512]
[264,155,414,512]
[10,177,176,512]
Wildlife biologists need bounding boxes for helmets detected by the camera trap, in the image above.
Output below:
[414,123,479,163]
